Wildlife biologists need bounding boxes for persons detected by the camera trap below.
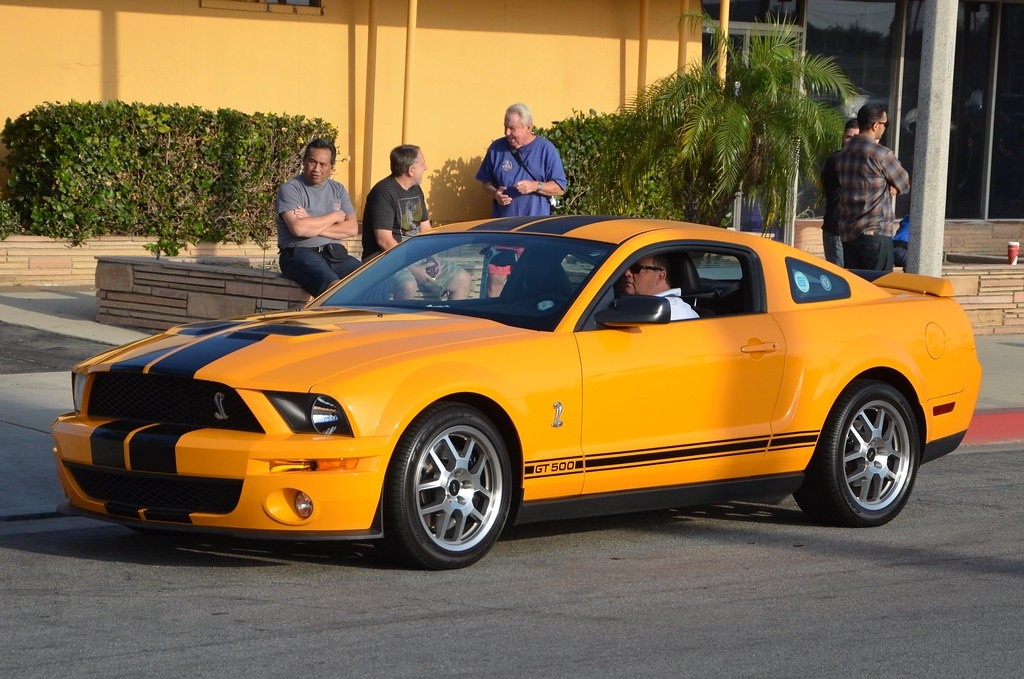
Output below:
[363,144,474,302]
[476,102,565,296]
[623,255,701,323]
[821,105,910,279]
[276,139,391,305]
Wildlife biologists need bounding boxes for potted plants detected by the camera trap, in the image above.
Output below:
[794,208,825,260]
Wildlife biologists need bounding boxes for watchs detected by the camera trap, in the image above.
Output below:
[537,180,543,192]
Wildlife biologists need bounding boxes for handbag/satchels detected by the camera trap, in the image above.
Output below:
[322,243,348,263]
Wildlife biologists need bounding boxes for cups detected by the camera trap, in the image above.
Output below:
[425,262,439,280]
[1008,242,1019,265]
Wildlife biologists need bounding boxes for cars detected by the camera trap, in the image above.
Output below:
[51,216,984,572]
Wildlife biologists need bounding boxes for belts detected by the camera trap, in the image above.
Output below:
[307,245,329,252]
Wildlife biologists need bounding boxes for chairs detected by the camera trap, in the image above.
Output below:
[659,254,699,308]
[518,258,568,301]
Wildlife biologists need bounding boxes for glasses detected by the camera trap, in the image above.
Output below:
[628,263,664,274]
[871,121,889,128]
